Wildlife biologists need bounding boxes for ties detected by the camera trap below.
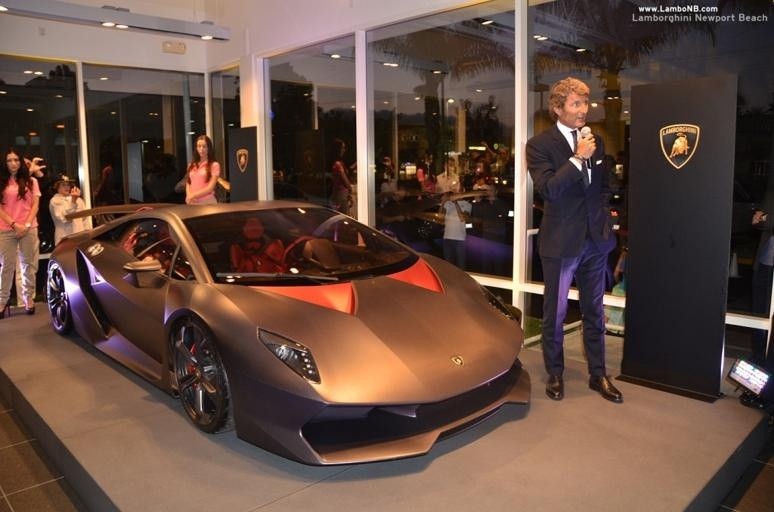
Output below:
[569,130,588,188]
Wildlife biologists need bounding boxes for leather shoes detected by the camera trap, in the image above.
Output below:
[588,375,623,403]
[545,373,564,400]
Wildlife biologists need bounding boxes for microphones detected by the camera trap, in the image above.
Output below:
[580,125,596,171]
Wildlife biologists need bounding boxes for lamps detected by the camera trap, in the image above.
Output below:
[0,0,230,41]
[724,355,774,409]
[322,46,449,75]
[472,12,597,56]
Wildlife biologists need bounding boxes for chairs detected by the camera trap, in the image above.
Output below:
[230,217,289,274]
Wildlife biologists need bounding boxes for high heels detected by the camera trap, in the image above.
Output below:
[0,299,11,319]
[22,298,34,314]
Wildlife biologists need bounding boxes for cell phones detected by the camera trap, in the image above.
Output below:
[68,179,76,193]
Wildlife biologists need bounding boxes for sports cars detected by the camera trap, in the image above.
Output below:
[375,190,546,284]
[46,197,535,469]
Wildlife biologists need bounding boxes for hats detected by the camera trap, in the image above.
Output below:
[54,174,76,184]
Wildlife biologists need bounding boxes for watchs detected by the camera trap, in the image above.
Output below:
[24,223,32,228]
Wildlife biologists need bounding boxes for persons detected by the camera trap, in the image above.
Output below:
[326,138,511,272]
[23,157,49,190]
[525,77,621,402]
[739,189,774,409]
[185,136,220,205]
[0,149,42,318]
[49,175,90,246]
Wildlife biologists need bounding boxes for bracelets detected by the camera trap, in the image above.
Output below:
[574,154,585,161]
[11,222,16,228]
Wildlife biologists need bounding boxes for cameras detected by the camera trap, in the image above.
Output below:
[37,159,48,166]
